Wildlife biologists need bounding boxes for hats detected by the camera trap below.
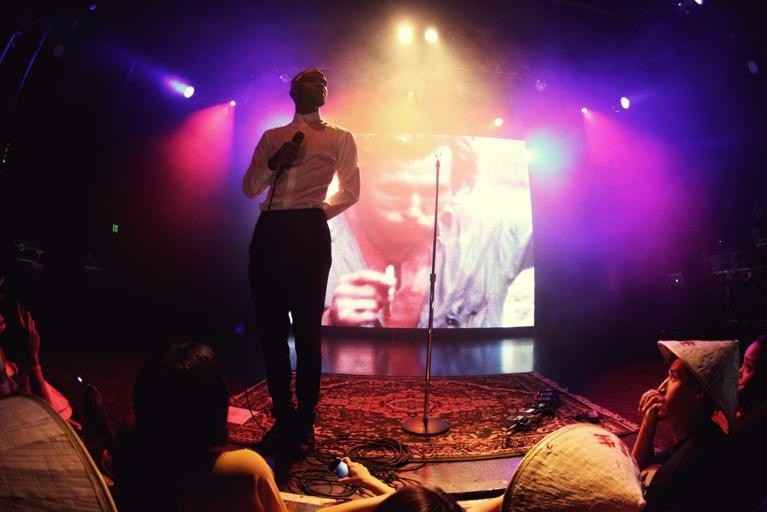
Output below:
[501,420,646,512]
[0,394,120,511]
[657,339,740,421]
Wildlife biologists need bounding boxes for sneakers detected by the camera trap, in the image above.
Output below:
[257,420,316,458]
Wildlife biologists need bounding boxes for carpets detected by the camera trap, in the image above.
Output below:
[225,372,639,465]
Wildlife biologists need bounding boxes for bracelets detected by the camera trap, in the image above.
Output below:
[28,365,42,372]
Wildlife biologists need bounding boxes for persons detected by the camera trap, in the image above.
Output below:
[468,420,648,511]
[733,330,766,511]
[621,338,759,511]
[102,334,294,512]
[240,67,362,465]
[0,297,78,425]
[305,134,534,329]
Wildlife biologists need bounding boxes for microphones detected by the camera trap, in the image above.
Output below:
[275,131,305,177]
